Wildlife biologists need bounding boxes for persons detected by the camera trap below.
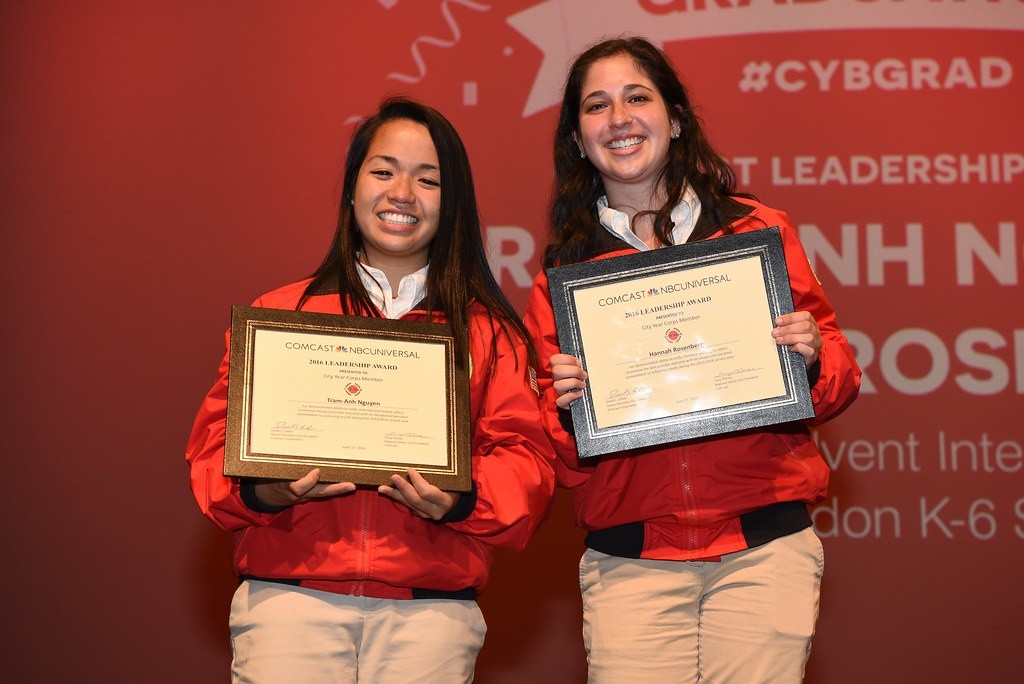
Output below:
[186,96,558,684]
[522,36,863,684]
